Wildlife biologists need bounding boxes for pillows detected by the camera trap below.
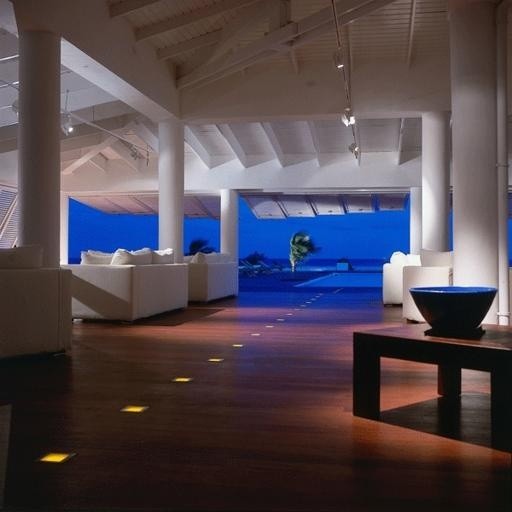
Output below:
[389,248,453,268]
[185,248,235,263]
[79,244,175,271]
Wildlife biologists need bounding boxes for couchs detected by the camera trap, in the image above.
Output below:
[1,258,238,361]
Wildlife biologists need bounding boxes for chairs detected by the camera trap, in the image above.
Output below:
[382,263,455,326]
